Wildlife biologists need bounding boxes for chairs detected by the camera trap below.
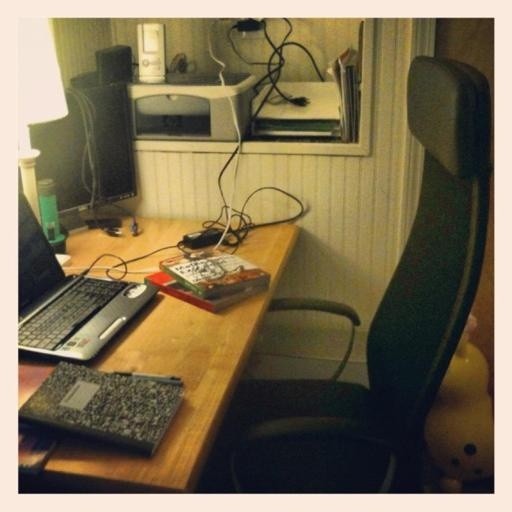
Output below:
[197,56,493,492]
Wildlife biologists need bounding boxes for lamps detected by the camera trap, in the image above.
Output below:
[19,17,70,228]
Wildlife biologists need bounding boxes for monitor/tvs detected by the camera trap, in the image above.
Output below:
[25,84,138,233]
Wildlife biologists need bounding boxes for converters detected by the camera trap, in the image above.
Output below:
[237,21,260,31]
[182,228,221,249]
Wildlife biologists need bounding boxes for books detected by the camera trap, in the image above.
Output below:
[19,360,184,457]
[159,248,269,297]
[143,270,268,313]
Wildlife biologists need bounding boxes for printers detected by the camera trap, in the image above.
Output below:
[128,71,256,142]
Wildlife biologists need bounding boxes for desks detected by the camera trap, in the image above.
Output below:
[19,214,302,492]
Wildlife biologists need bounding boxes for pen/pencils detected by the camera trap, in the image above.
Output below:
[115,372,181,381]
[130,375,182,387]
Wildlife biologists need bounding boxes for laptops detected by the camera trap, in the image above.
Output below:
[19,188,159,364]
